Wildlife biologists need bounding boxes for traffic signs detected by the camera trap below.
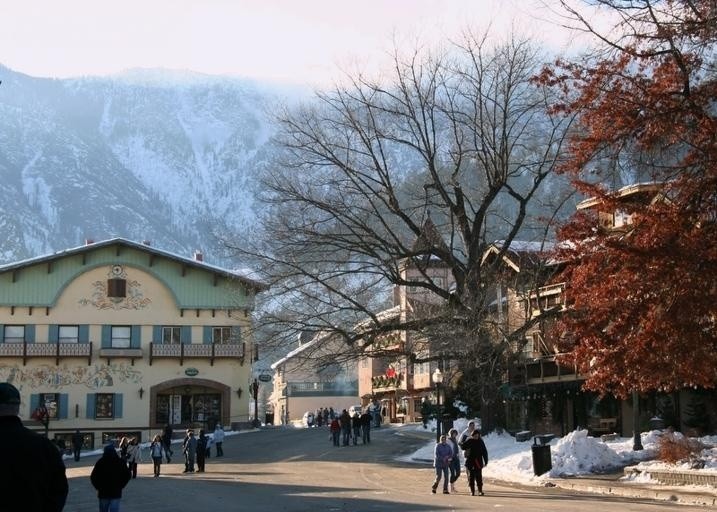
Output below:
[432,368,443,443]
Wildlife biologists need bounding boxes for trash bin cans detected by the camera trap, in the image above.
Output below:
[531,435,551,476]
[266,412,273,426]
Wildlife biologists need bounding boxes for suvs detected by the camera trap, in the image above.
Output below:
[315,409,341,425]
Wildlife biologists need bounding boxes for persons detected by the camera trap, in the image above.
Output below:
[90,445,131,512]
[31,403,47,418]
[458,421,474,491]
[119,424,225,479]
[460,430,488,496]
[446,428,460,492]
[305,406,373,447]
[432,435,452,494]
[72,429,83,461]
[0,383,69,512]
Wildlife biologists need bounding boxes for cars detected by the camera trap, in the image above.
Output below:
[349,405,362,419]
